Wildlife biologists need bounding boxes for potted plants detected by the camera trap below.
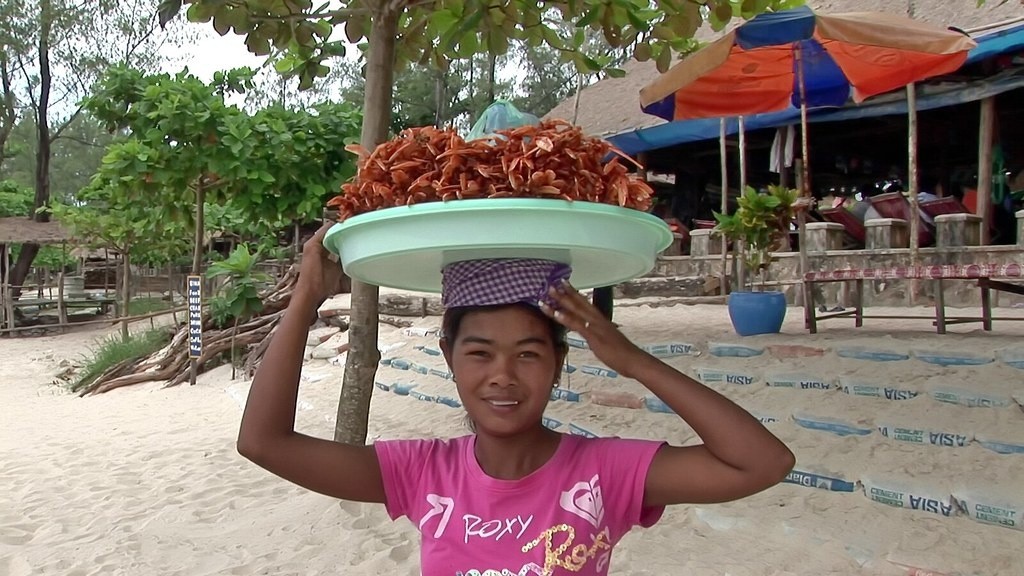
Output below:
[705,184,803,333]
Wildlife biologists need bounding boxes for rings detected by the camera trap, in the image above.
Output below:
[585,321,590,330]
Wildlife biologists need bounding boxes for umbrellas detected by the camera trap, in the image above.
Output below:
[637,4,977,198]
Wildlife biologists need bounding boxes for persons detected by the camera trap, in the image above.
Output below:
[236,222,797,576]
[959,179,993,230]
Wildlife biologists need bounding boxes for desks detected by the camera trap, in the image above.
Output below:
[800,260,1024,332]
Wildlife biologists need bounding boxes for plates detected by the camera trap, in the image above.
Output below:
[321,197,674,294]
